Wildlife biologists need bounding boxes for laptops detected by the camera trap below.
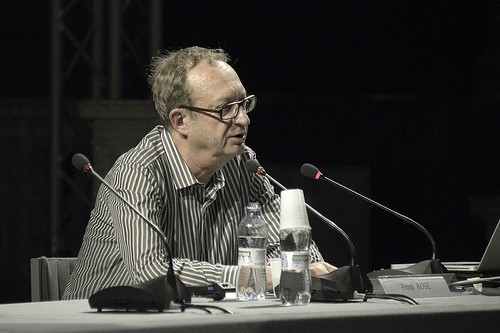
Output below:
[440,218,500,274]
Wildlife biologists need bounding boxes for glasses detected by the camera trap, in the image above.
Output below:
[179,95,257,120]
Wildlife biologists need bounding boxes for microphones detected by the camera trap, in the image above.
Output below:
[299,162,444,273]
[71,153,192,310]
[244,159,374,302]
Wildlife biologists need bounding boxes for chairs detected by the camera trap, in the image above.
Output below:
[31,257,79,301]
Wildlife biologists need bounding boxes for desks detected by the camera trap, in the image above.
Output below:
[0,277,500,333]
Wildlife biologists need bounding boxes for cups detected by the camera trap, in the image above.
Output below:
[278,189,312,229]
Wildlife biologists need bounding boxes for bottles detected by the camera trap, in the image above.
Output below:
[279,227,311,306]
[236,203,269,301]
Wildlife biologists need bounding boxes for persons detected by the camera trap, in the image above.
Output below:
[61,46,340,301]
